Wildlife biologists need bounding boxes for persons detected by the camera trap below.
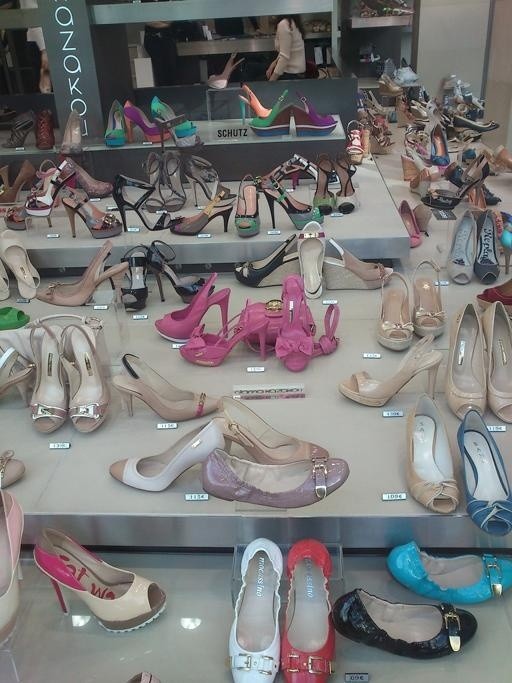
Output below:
[142,0,180,88]
[18,1,53,114]
[267,15,309,81]
[212,16,263,62]
